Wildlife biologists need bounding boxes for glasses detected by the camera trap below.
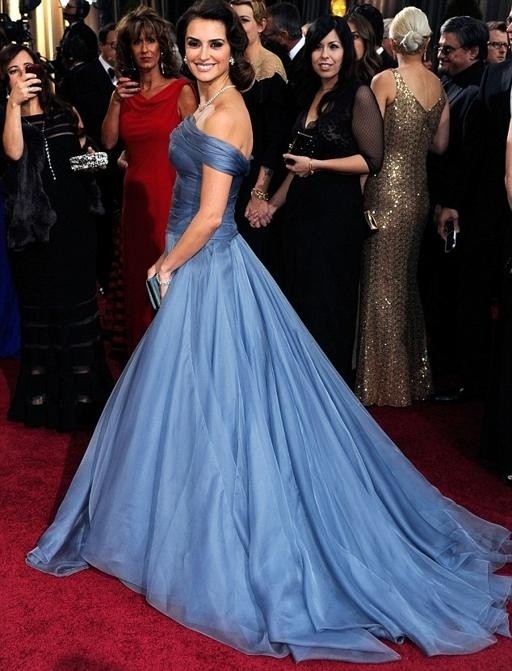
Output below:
[487,41,508,50]
[262,31,277,39]
[434,43,464,55]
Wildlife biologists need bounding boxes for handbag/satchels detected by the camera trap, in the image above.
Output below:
[284,131,345,165]
[68,151,109,172]
[145,273,161,311]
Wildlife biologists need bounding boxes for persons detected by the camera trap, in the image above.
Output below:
[141,3,273,606]
[0,0,511,488]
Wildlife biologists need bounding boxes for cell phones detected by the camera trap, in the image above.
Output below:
[127,67,140,88]
[26,62,43,81]
[444,220,457,253]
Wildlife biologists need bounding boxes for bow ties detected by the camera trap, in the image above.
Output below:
[108,68,117,80]
